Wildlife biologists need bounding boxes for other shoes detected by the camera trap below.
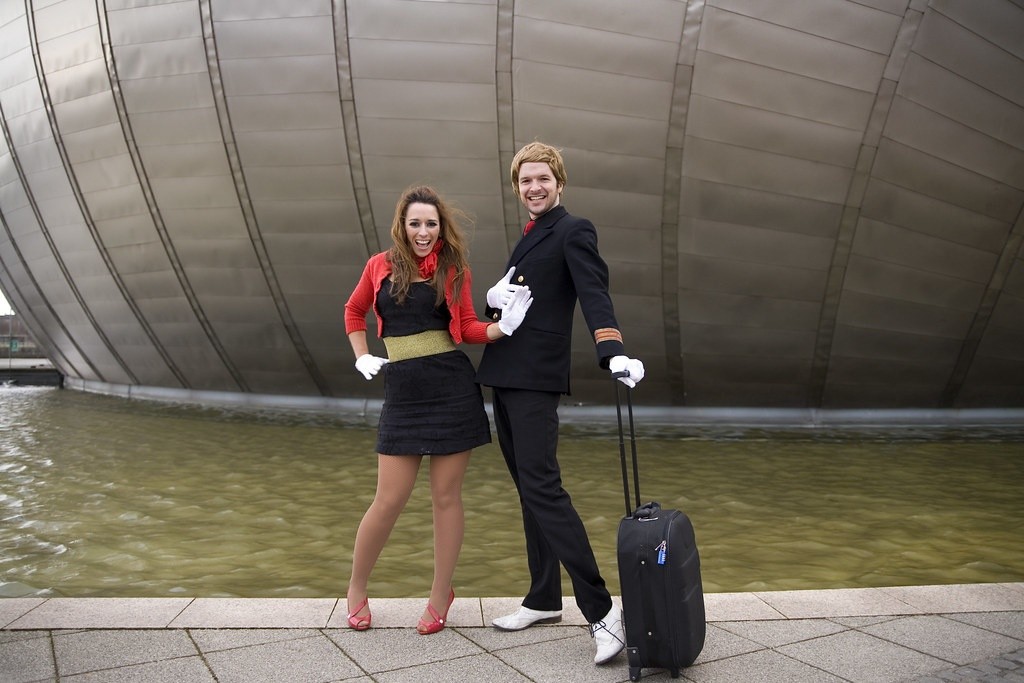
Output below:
[589,599,626,665]
[492,605,563,631]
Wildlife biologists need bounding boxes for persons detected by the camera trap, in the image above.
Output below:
[344,186,533,635]
[474,141,646,662]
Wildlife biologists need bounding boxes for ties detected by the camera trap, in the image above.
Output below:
[523,220,535,238]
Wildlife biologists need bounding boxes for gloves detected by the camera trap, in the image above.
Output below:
[609,355,645,389]
[486,266,525,310]
[498,285,533,337]
[355,353,391,380]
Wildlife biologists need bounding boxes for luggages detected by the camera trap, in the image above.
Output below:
[608,371,707,683]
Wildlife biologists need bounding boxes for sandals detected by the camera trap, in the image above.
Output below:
[346,595,373,632]
[416,587,456,635]
